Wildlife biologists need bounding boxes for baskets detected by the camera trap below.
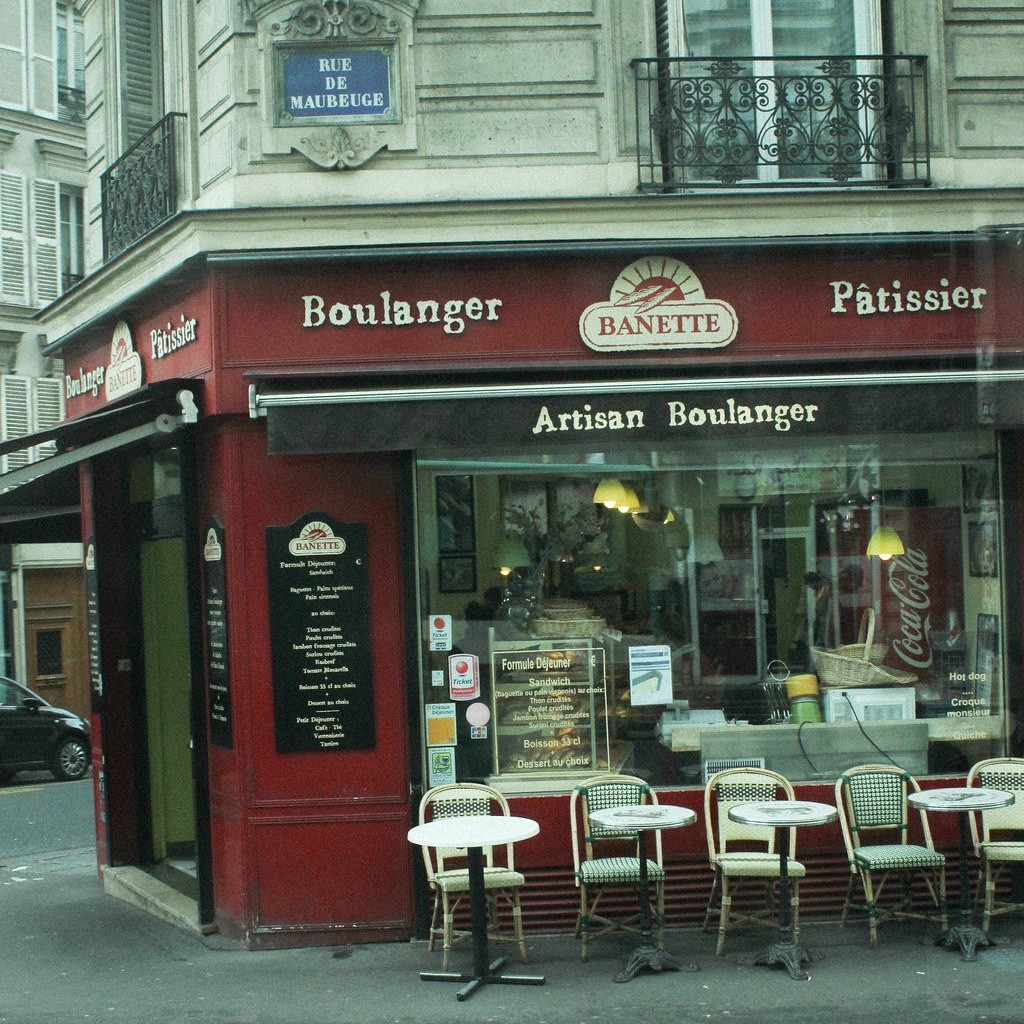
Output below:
[810,607,918,688]
[533,600,607,638]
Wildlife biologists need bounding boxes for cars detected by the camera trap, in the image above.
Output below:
[0,676,91,788]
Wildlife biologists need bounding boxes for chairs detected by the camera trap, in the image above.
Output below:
[834,764,951,950]
[966,757,1024,934]
[418,781,530,974]
[570,774,665,964]
[701,765,805,956]
[643,566,691,645]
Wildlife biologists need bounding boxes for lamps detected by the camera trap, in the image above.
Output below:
[865,464,904,561]
[736,468,758,501]
[592,478,676,525]
[490,481,532,569]
[687,470,725,565]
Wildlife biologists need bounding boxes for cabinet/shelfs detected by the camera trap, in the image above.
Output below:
[486,626,627,773]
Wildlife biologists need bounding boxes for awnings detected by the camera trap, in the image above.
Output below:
[0,378,205,477]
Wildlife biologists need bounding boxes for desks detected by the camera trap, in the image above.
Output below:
[907,785,1016,962]
[407,815,545,1002]
[729,800,839,982]
[587,804,701,983]
[699,597,768,681]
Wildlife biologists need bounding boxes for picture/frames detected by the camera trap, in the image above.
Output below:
[974,613,999,715]
[438,554,478,594]
[435,475,477,555]
[968,519,999,578]
[961,452,1000,514]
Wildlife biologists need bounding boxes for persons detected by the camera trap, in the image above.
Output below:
[642,617,729,776]
[697,560,726,597]
[456,585,504,760]
